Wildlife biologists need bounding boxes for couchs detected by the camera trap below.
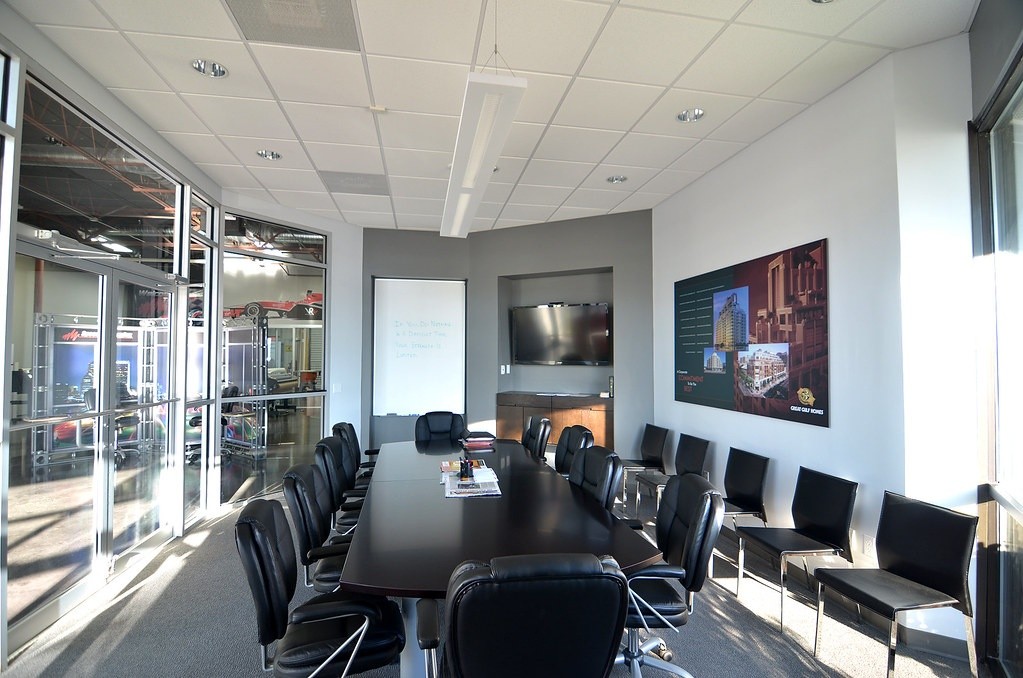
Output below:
[268,368,299,413]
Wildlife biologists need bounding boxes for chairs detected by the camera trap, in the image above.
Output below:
[613,473,726,678]
[314,437,368,534]
[814,491,979,678]
[282,463,355,594]
[234,499,406,678]
[332,421,380,488]
[635,433,711,515]
[522,415,551,462]
[571,446,624,512]
[185,385,240,464]
[84,388,144,471]
[555,425,594,478]
[736,465,864,633]
[707,447,777,578]
[621,422,669,512]
[268,379,297,413]
[415,411,464,441]
[415,552,630,678]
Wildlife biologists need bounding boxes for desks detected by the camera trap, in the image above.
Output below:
[340,439,663,678]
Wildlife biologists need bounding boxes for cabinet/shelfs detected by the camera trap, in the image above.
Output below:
[497,391,614,452]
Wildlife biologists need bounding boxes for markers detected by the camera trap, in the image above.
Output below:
[459,456,468,462]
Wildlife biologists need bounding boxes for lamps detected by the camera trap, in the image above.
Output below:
[439,72,528,238]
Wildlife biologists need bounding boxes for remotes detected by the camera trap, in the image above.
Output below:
[609,376,614,398]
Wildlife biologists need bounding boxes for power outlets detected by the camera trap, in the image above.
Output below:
[849,528,855,549]
[703,470,710,481]
[864,534,876,557]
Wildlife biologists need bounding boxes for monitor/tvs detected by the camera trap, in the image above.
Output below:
[512,302,612,366]
